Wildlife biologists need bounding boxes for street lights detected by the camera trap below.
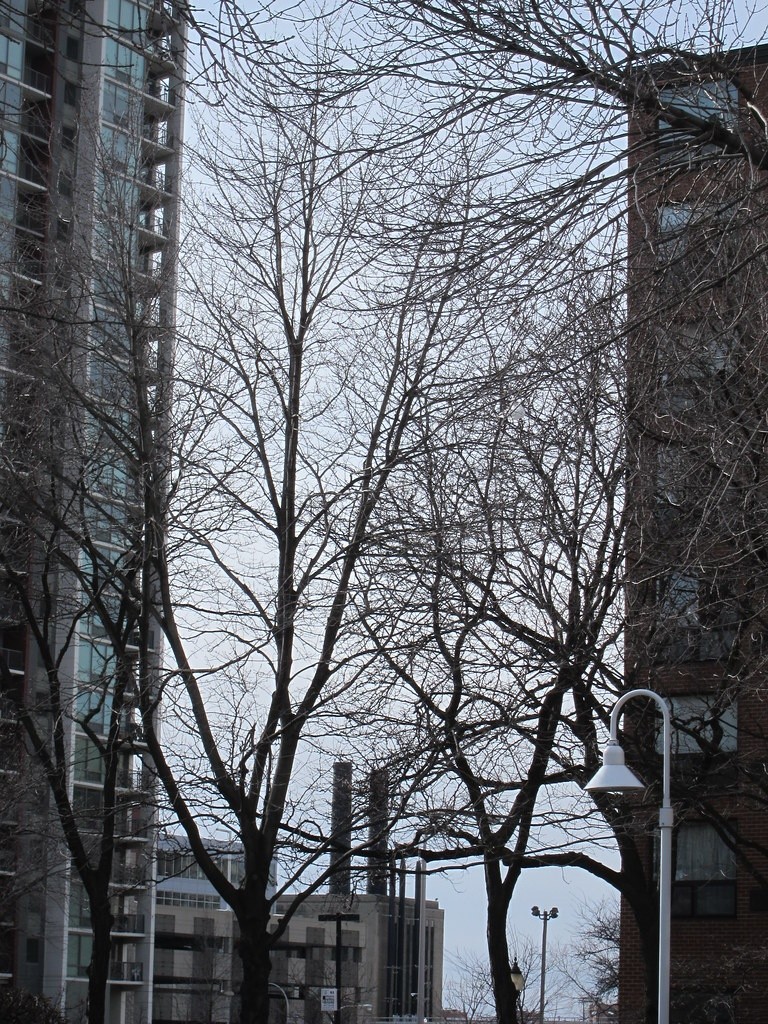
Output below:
[318,911,360,1024]
[531,905,559,1024]
[584,689,675,1024]
